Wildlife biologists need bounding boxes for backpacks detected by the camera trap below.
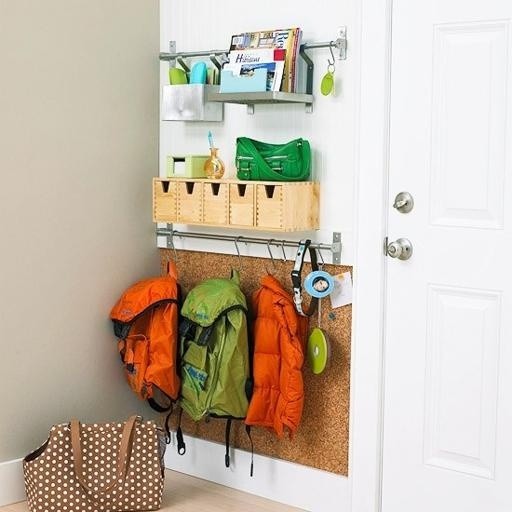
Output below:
[109,258,180,412]
[174,266,252,421]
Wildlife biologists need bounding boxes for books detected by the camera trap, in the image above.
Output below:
[222,26,302,93]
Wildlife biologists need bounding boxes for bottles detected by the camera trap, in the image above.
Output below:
[203,147,225,179]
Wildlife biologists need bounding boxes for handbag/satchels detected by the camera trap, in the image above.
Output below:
[236,137,312,182]
[23,414,167,512]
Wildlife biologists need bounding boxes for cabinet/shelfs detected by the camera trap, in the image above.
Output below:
[159,27,347,121]
[152,155,320,233]
